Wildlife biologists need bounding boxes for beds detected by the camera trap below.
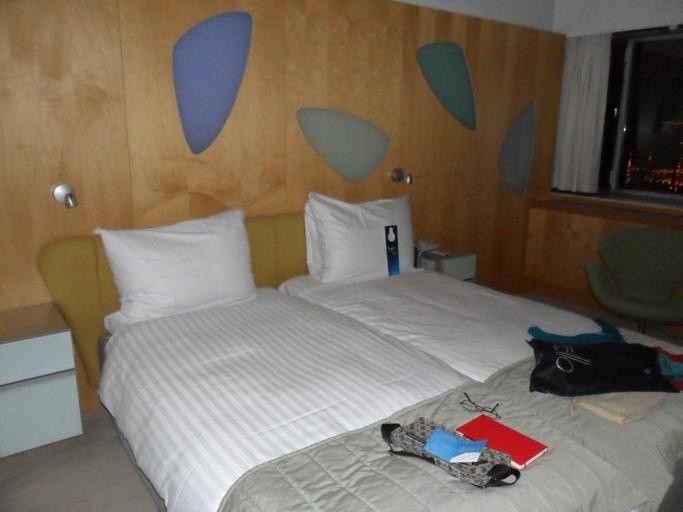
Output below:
[37,212,683,512]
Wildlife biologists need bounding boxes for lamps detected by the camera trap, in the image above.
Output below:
[50,181,78,209]
[391,168,412,185]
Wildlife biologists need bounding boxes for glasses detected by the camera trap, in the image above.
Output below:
[459,391,502,421]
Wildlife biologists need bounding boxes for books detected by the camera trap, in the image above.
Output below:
[457,412,550,472]
[574,391,668,426]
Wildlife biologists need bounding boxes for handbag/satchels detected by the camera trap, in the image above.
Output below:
[524,322,673,396]
[380,418,521,488]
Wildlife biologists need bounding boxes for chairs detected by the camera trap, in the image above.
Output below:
[584,228,683,335]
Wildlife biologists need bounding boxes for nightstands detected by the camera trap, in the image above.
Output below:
[420,247,477,283]
[0,302,84,462]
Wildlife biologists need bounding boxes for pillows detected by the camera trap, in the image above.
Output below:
[304,201,322,280]
[308,191,424,288]
[92,208,257,336]
